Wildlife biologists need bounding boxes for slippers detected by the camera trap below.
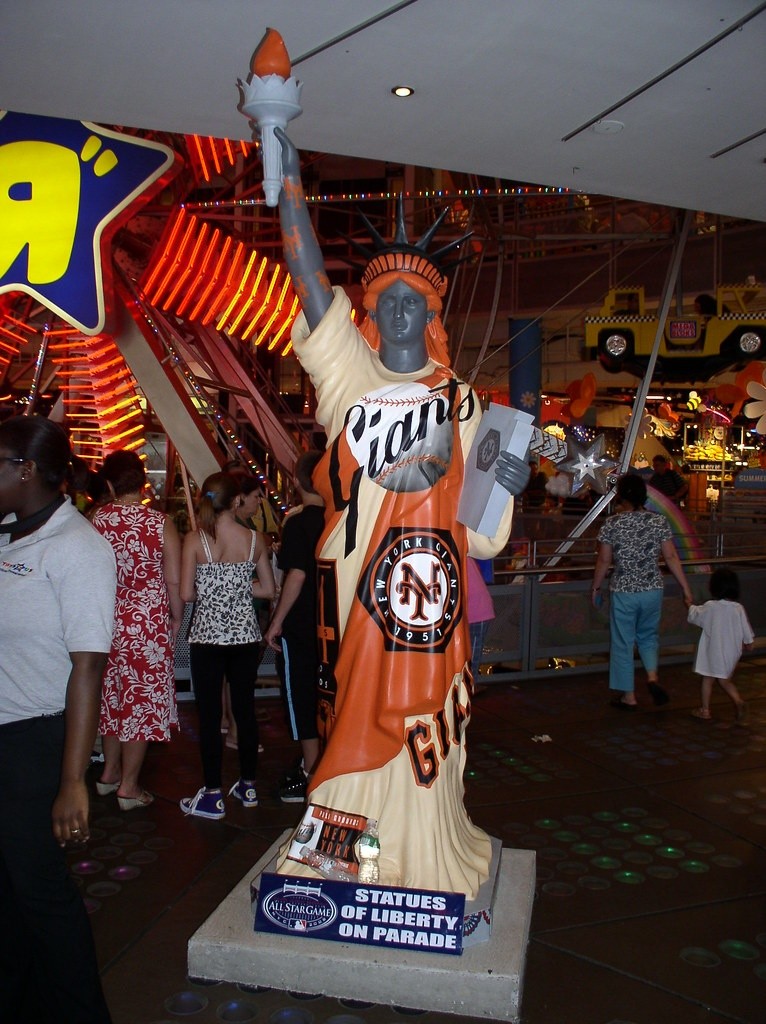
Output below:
[608,695,640,712]
[225,744,265,752]
[647,681,672,706]
[221,727,230,734]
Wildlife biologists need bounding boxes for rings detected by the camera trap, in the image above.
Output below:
[71,829,79,833]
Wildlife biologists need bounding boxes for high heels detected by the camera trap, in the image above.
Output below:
[117,785,155,810]
[95,779,122,795]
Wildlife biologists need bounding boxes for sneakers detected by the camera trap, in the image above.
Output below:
[228,776,259,808]
[180,787,227,819]
[278,758,305,782]
[278,774,309,803]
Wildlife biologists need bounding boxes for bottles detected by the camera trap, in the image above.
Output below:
[358,819,380,884]
[299,846,358,883]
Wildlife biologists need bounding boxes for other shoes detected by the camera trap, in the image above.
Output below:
[90,749,105,763]
[471,685,490,696]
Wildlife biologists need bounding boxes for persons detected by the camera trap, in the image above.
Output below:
[521,461,546,528]
[684,569,755,721]
[591,472,692,711]
[265,450,326,803]
[249,117,532,899]
[180,460,281,820]
[0,417,117,1024]
[650,454,688,500]
[89,450,185,811]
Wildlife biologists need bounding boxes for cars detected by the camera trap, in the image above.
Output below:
[583,285,765,364]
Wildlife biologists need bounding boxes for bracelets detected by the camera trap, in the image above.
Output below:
[592,587,600,592]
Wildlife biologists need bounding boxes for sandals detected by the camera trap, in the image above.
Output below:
[691,706,712,719]
[737,701,750,720]
